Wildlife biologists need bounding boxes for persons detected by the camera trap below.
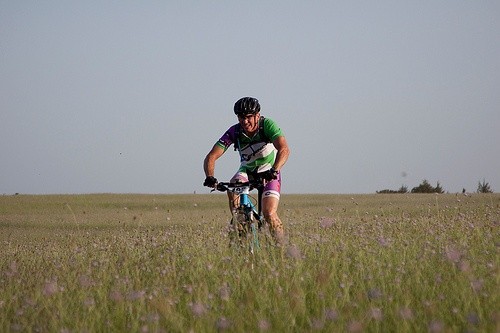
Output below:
[203,96,290,244]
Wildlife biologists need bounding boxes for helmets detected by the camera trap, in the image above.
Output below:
[234,97,260,116]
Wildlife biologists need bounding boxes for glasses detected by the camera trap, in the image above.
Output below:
[238,113,256,121]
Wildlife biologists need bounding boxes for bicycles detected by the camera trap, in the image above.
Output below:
[203,172,279,272]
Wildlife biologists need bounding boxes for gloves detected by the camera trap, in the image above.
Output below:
[205,176,218,188]
[261,169,278,182]
